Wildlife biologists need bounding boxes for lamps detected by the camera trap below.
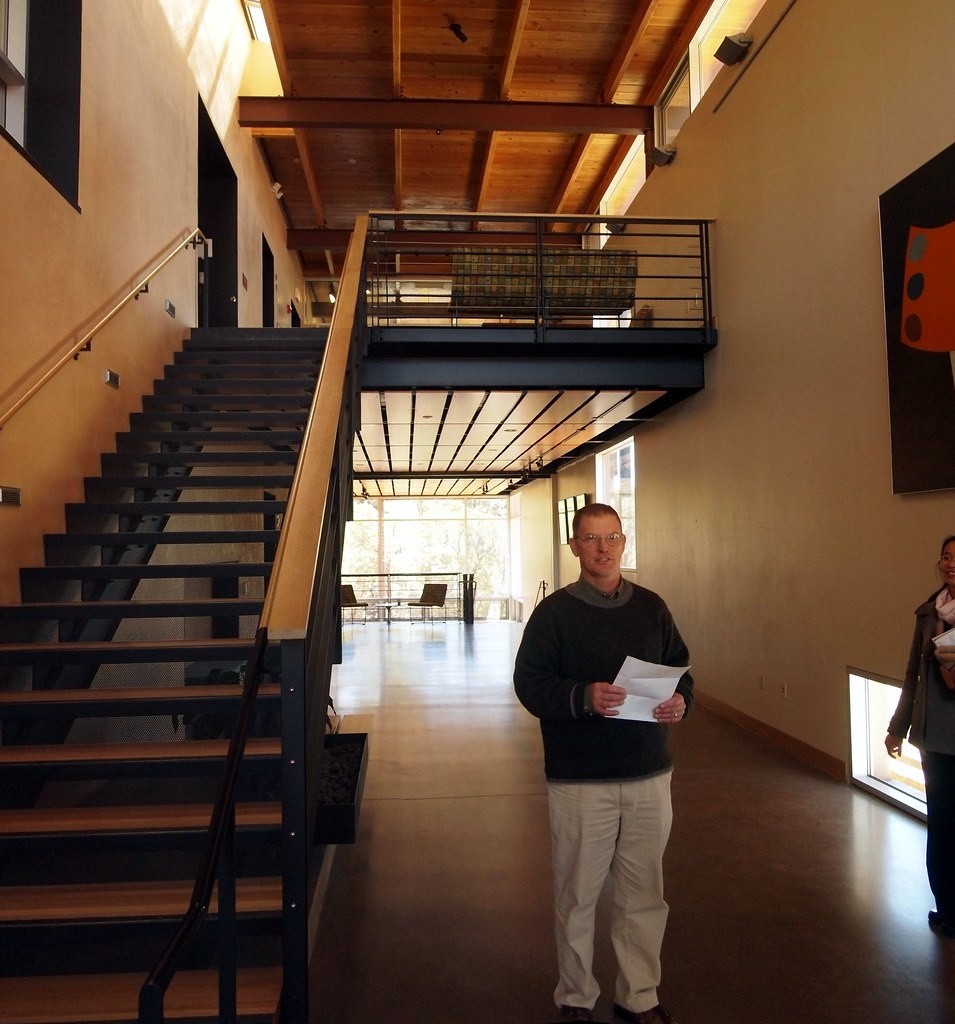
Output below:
[649,144,677,167]
[605,222,625,234]
[713,32,754,67]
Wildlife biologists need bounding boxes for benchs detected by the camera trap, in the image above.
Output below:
[449,247,638,326]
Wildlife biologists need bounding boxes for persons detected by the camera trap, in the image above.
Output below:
[884,534,955,941]
[509,503,695,1024]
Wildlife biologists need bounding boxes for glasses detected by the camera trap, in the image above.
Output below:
[573,533,625,547]
[938,555,955,563]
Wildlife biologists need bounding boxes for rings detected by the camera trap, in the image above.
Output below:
[674,712,678,717]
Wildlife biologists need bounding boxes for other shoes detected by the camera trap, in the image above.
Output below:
[614,1003,682,1024]
[560,1005,592,1022]
[929,912,955,939]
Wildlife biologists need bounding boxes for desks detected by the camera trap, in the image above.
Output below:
[375,604,399,624]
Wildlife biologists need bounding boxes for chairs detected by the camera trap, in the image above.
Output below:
[339,585,368,625]
[407,583,448,625]
